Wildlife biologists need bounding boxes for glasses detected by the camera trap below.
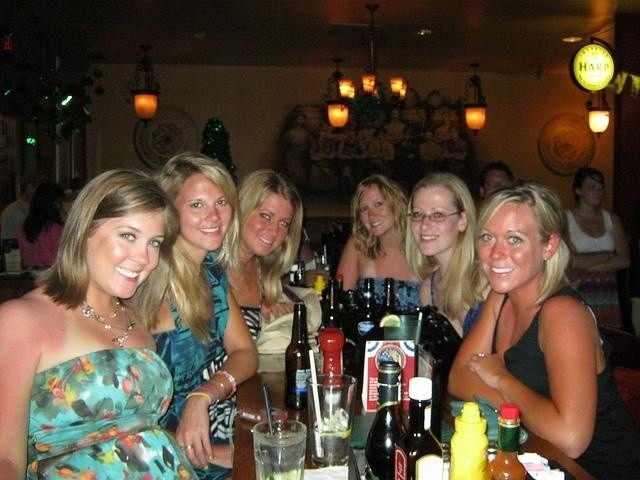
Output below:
[410,211,460,222]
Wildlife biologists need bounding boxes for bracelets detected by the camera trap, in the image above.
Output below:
[185,371,237,406]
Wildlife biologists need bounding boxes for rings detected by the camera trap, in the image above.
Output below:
[184,445,193,452]
[478,353,485,358]
[180,446,184,450]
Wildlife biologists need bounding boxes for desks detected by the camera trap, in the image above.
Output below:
[0,272,35,303]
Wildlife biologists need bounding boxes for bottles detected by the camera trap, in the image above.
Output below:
[320,328,345,383]
[290,261,306,288]
[394,375,444,480]
[1,40,87,198]
[488,403,527,479]
[286,302,310,409]
[451,402,487,480]
[314,275,395,351]
[364,362,407,479]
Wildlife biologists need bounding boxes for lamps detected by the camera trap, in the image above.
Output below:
[128,45,161,129]
[339,3,408,120]
[587,90,612,139]
[464,63,490,137]
[324,58,349,131]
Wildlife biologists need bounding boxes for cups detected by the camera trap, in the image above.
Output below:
[306,375,358,468]
[315,256,326,269]
[253,421,306,480]
[382,315,420,340]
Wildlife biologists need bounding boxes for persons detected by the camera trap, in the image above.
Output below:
[447,180,640,479]
[562,167,632,331]
[202,168,304,446]
[0,180,36,240]
[404,171,493,342]
[15,181,66,274]
[288,240,332,289]
[336,174,428,315]
[473,161,515,215]
[0,167,201,480]
[121,151,258,480]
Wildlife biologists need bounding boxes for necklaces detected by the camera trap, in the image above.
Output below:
[80,297,136,348]
